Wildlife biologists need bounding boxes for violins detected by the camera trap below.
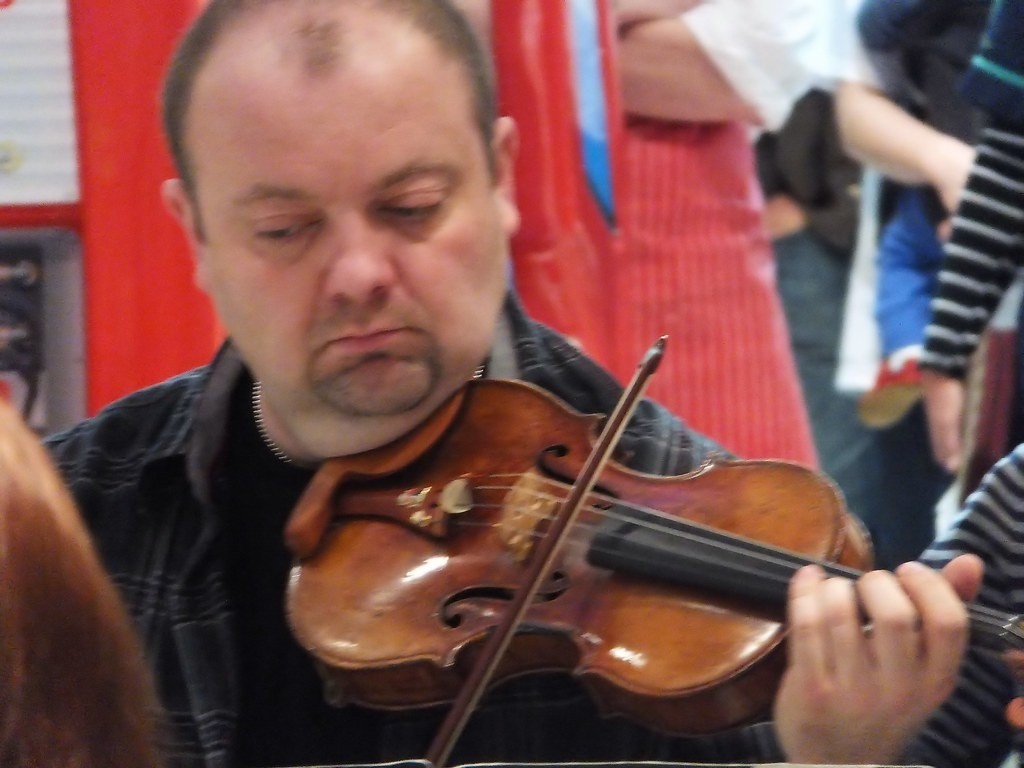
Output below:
[284,373,1024,741]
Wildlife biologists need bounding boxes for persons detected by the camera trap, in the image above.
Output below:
[0,398,168,768]
[36,1,984,768]
[448,1,1024,768]
